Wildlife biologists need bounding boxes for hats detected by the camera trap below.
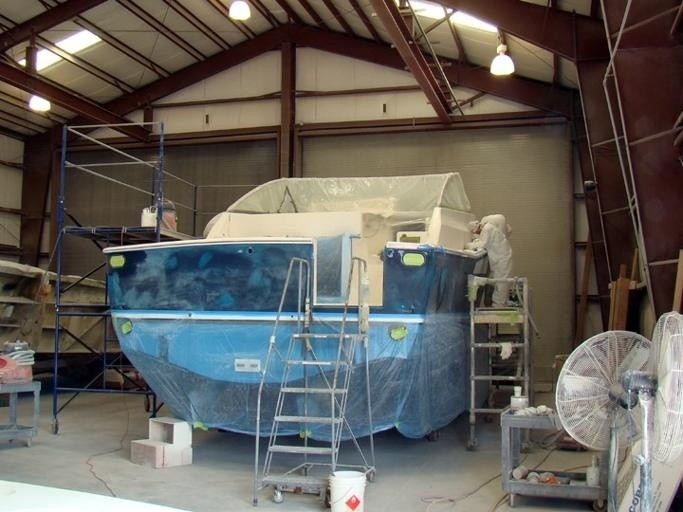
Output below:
[467,221,479,233]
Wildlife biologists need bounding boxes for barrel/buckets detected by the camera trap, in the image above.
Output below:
[326,471,367,512]
[160,198,178,232]
[140,205,158,228]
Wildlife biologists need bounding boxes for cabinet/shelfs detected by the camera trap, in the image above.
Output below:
[0,296,77,329]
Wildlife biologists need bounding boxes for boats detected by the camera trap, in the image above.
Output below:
[103,172,493,441]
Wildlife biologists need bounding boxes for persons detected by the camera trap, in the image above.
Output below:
[465,222,513,307]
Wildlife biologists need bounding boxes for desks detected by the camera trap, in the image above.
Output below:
[499,405,609,511]
[1,382,41,449]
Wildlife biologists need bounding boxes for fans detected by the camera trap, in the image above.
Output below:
[554,310,682,512]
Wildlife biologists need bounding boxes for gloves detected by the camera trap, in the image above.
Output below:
[465,243,474,250]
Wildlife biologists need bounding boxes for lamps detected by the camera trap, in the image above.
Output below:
[228,0,251,21]
[28,95,52,111]
[489,27,517,77]
